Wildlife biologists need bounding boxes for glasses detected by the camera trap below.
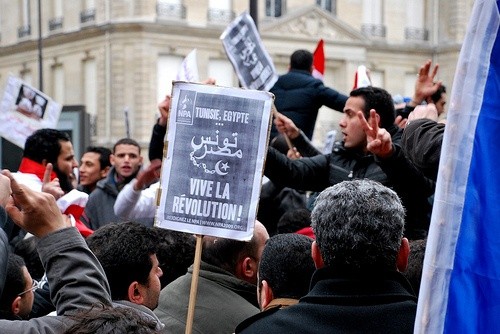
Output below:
[18,279,39,295]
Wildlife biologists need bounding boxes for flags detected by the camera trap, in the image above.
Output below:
[413,1,500,334]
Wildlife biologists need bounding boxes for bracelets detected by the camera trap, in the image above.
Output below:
[134,187,142,190]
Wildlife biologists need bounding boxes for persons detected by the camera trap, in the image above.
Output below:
[0,60,448,334]
[267,49,349,144]
